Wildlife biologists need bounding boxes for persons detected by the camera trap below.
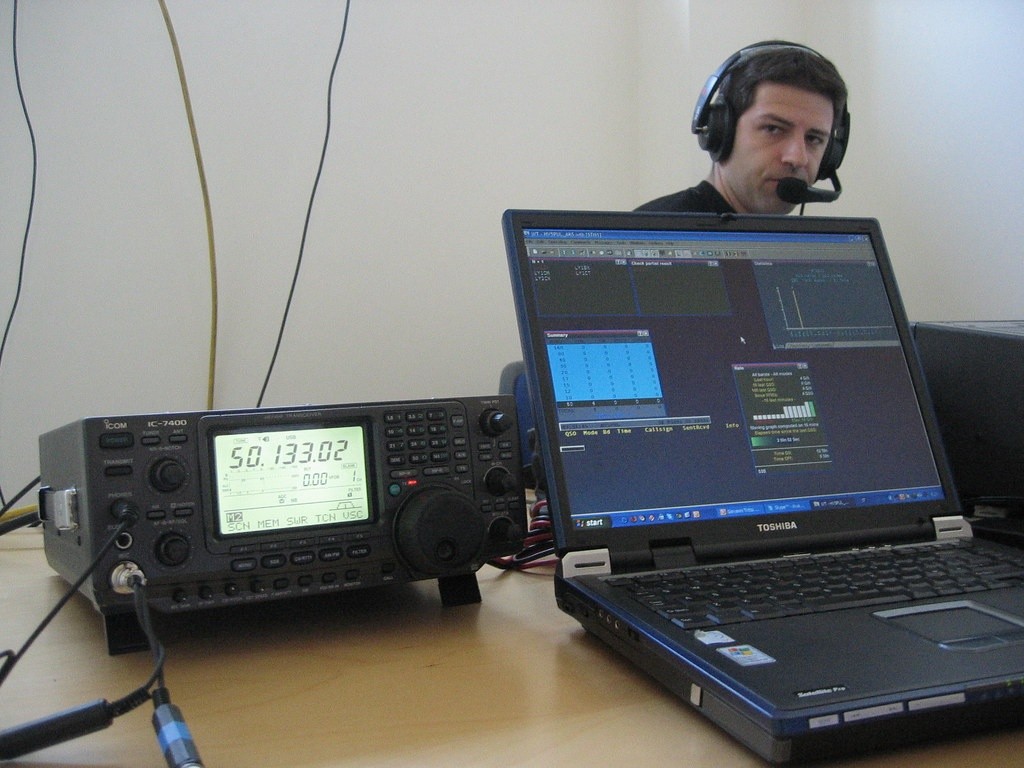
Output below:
[631,48,848,213]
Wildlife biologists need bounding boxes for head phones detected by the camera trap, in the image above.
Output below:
[690,41,851,180]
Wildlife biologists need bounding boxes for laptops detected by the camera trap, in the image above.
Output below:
[502,208,1023,767]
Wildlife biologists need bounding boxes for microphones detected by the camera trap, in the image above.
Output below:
[776,172,842,205]
